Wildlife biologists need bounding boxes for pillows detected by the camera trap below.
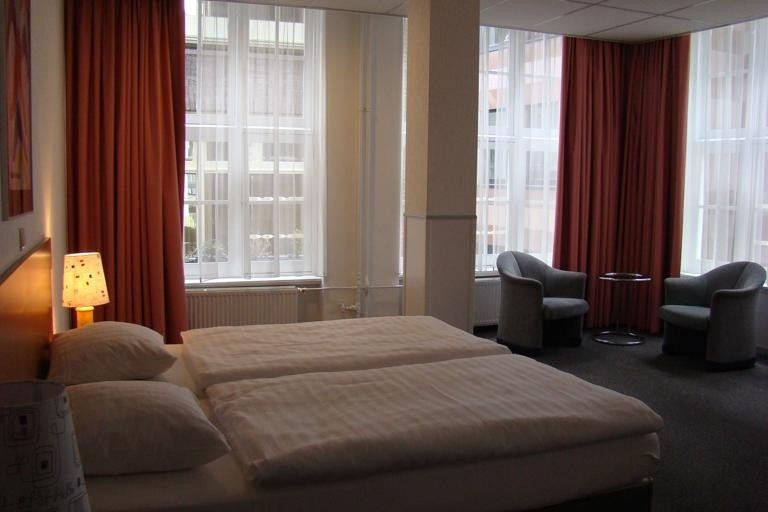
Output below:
[45,321,229,511]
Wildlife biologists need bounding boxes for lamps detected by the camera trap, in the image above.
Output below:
[60,245,110,333]
[0,380,91,512]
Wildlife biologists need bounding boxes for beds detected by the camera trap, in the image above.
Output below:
[1,233,729,511]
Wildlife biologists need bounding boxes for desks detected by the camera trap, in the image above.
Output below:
[596,271,650,347]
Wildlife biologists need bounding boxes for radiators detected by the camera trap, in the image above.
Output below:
[187,286,301,330]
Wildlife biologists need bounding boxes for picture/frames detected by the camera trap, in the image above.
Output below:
[0,0,33,221]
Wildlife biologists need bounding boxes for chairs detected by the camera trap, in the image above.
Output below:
[497,250,588,354]
[663,262,768,369]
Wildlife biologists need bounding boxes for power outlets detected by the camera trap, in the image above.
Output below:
[19,228,30,252]
